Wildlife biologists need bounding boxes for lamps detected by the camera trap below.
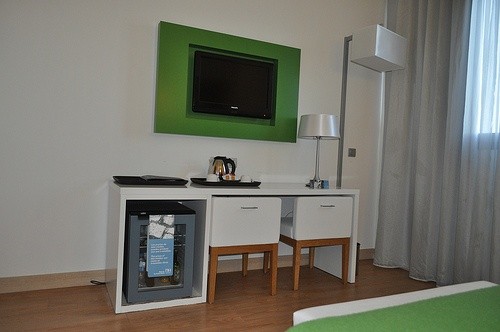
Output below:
[298,114,341,189]
[336,24,407,190]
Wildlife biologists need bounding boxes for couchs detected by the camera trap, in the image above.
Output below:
[287,280,500,332]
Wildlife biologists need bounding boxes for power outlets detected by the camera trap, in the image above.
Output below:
[348,147,356,158]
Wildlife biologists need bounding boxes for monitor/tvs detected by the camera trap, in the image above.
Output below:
[192,50,274,119]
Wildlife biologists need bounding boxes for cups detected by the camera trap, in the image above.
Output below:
[240,175,253,182]
[207,174,219,182]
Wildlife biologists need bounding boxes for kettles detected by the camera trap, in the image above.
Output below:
[212,156,235,181]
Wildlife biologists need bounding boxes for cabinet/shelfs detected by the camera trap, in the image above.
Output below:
[106,179,360,314]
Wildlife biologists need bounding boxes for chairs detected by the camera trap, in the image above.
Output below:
[263,197,353,291]
[208,196,281,305]
